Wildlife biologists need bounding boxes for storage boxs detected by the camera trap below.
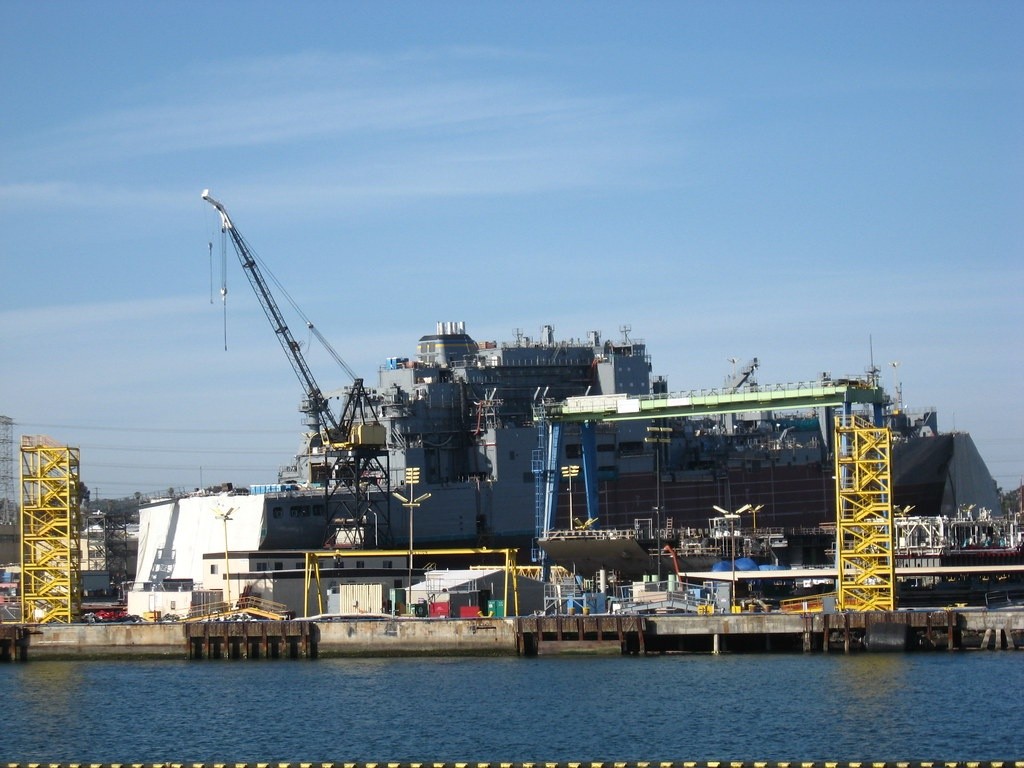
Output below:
[0,572,13,583]
[488,600,503,617]
[583,593,606,613]
[461,606,479,617]
[429,602,449,618]
[250,483,296,493]
[410,604,427,617]
[567,599,583,613]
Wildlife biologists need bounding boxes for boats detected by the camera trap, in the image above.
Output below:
[859,506,1024,600]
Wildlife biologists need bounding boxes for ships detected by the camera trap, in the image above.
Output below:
[124,189,1003,614]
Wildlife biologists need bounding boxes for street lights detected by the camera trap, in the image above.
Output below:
[393,492,431,614]
[645,426,672,583]
[713,504,753,607]
[212,505,239,612]
